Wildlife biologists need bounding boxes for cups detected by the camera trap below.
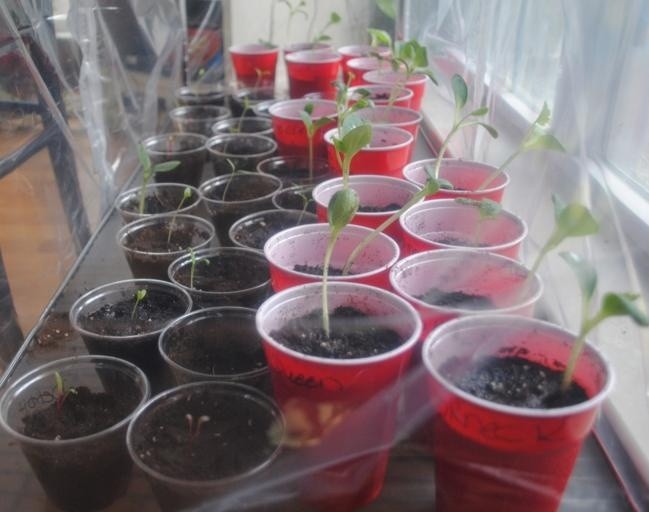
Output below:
[255,281,424,510]
[0,353,149,512]
[66,277,195,404]
[422,317,612,512]
[229,41,529,291]
[158,306,272,393]
[114,80,334,315]
[392,249,547,338]
[127,380,285,512]
